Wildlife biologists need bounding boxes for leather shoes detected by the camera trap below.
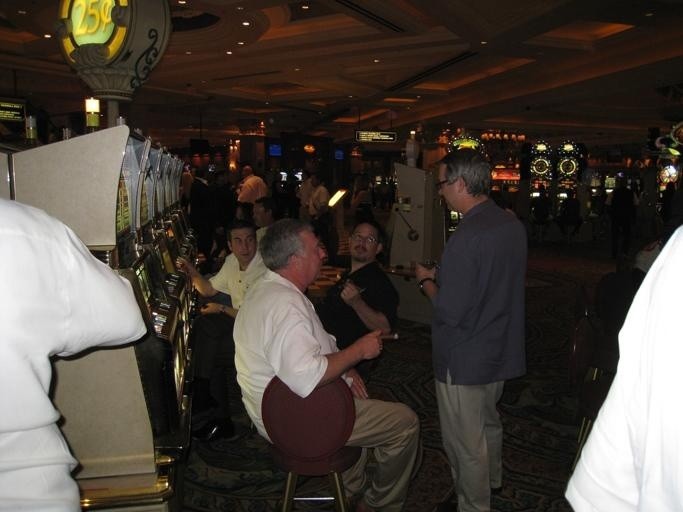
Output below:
[190,418,238,443]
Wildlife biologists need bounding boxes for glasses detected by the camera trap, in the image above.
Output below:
[351,233,376,243]
[435,180,447,190]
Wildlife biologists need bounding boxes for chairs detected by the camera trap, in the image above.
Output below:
[262,375,361,512]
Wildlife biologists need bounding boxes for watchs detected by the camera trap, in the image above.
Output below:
[418,276,436,293]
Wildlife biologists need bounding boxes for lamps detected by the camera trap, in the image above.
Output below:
[25,117,37,139]
[86,99,100,126]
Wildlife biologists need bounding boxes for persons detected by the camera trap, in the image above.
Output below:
[236,166,270,205]
[234,219,422,511]
[176,221,266,442]
[351,176,373,218]
[661,180,677,220]
[413,147,529,511]
[297,169,330,218]
[249,197,282,243]
[192,166,236,222]
[0,195,148,512]
[533,175,637,254]
[317,218,399,378]
[374,177,396,207]
